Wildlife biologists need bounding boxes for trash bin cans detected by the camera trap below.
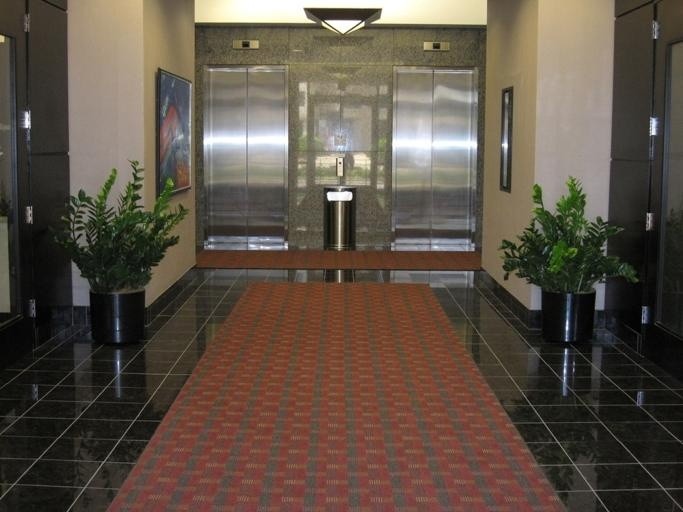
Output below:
[323,186,357,252]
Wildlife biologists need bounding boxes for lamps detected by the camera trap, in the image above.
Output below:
[303,8,382,36]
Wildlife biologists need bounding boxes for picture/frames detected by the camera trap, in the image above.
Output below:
[156,67,193,200]
[500,86,514,193]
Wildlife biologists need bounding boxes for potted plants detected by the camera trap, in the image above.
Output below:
[75,345,169,508]
[494,174,639,340]
[53,156,190,345]
[498,340,637,507]
